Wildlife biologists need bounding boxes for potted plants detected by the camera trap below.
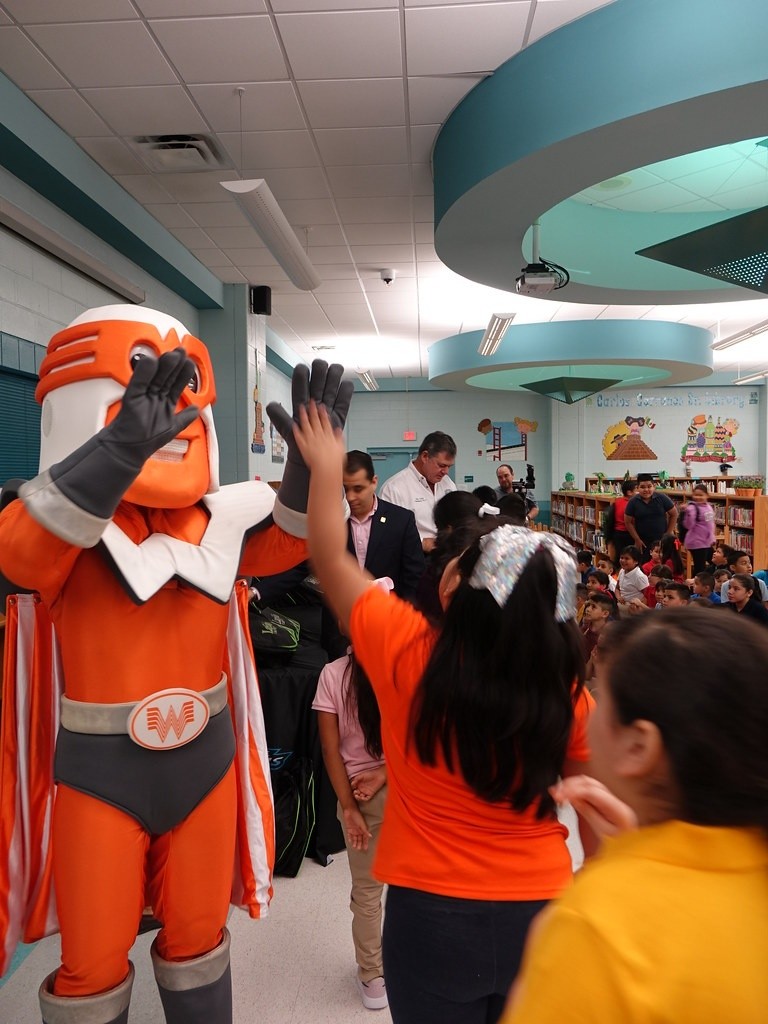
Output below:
[733,475,765,496]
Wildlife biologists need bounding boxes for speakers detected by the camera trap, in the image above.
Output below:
[253,286,271,316]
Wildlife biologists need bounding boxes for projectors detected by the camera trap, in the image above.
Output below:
[516,277,556,295]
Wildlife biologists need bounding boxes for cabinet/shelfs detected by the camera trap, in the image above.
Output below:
[653,489,768,580]
[585,476,736,493]
[551,490,620,569]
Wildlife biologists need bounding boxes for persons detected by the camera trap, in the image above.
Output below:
[0,301,353,1024]
[246,430,768,1024]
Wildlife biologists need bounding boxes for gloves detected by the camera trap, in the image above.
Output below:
[17,347,199,548]
[266,360,355,538]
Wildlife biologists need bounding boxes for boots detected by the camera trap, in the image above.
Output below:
[38,959,135,1024]
[150,926,233,1024]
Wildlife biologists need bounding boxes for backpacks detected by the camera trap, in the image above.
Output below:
[677,501,700,544]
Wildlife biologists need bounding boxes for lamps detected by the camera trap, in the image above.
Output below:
[219,87,321,291]
[478,312,517,356]
[354,368,380,391]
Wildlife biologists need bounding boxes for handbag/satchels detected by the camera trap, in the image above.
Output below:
[602,502,619,544]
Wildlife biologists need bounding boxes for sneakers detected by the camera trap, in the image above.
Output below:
[356,972,389,1008]
